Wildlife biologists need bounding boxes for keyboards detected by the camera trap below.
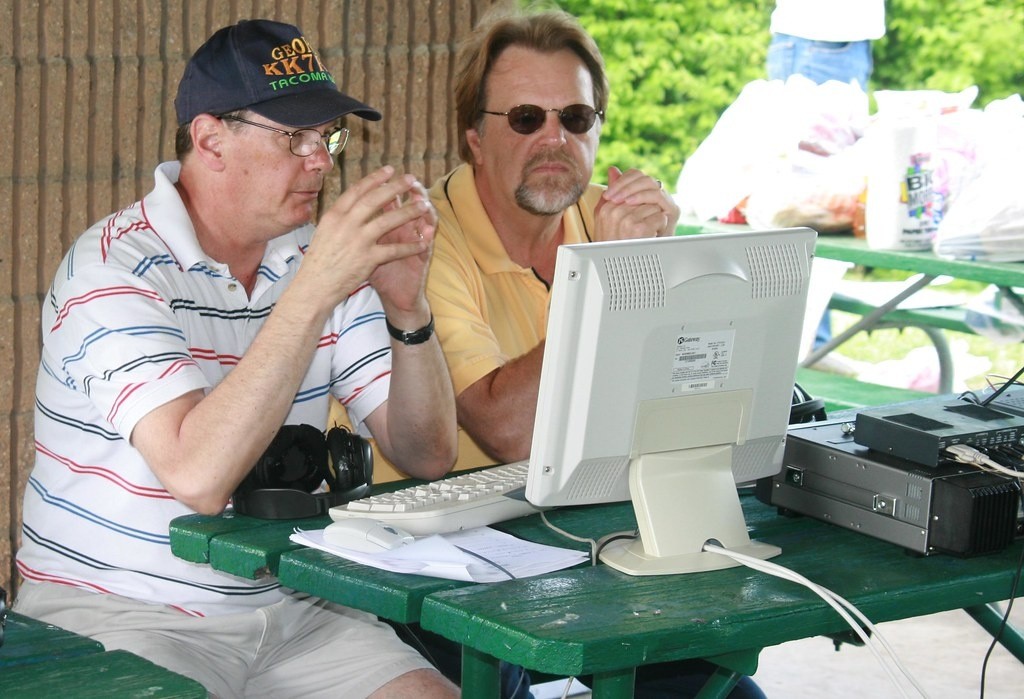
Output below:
[328,458,554,537]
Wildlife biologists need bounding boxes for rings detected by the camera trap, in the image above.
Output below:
[656,180,664,190]
[416,229,424,239]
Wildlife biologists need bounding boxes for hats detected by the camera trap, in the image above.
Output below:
[174,19,382,128]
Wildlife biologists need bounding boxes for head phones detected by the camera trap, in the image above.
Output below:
[788,383,826,425]
[233,422,374,520]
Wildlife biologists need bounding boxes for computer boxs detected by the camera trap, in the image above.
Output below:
[754,418,1024,558]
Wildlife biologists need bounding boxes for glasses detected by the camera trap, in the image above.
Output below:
[217,115,350,158]
[481,103,603,136]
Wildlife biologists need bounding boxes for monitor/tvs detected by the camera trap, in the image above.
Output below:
[524,226,818,580]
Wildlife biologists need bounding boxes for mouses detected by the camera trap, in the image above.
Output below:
[323,517,414,557]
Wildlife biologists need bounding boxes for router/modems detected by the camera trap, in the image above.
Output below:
[855,397,1024,469]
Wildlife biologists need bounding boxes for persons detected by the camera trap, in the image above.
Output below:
[327,11,771,699]
[766,0,886,351]
[10,18,462,697]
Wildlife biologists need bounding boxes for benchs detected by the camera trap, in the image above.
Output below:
[825,282,975,394]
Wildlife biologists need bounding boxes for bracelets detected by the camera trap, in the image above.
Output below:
[385,312,435,345]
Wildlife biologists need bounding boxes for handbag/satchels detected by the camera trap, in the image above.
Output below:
[675,75,1024,264]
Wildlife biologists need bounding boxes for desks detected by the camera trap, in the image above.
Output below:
[666,216,1023,392]
[169,394,1024,698]
